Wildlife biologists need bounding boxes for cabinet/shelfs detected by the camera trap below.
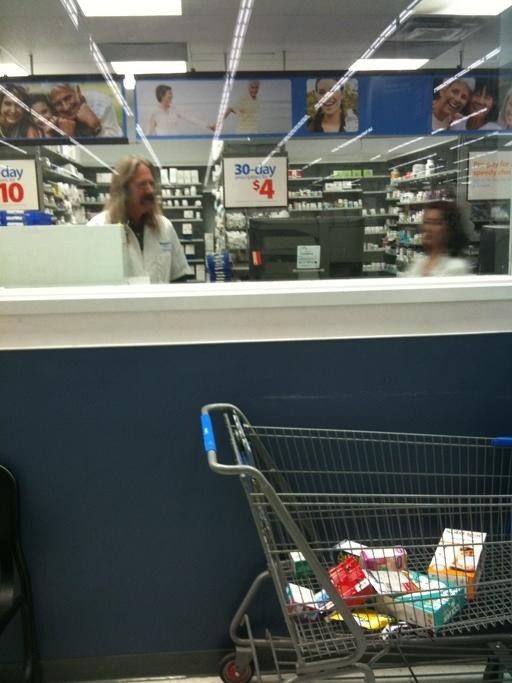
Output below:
[0,139,512,282]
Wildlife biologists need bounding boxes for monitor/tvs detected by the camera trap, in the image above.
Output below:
[248,217,365,280]
[289,208,362,217]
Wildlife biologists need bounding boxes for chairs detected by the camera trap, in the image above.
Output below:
[1,465,36,682]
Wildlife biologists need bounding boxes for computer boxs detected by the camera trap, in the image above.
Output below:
[478,224,509,273]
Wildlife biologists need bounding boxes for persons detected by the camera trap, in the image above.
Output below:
[22,90,57,139]
[46,83,124,138]
[447,76,501,131]
[307,78,358,132]
[141,83,211,134]
[211,78,265,134]
[88,153,197,285]
[0,79,27,138]
[400,199,471,278]
[498,87,512,131]
[430,74,476,131]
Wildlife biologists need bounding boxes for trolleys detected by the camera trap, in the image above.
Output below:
[200,402,512,682]
